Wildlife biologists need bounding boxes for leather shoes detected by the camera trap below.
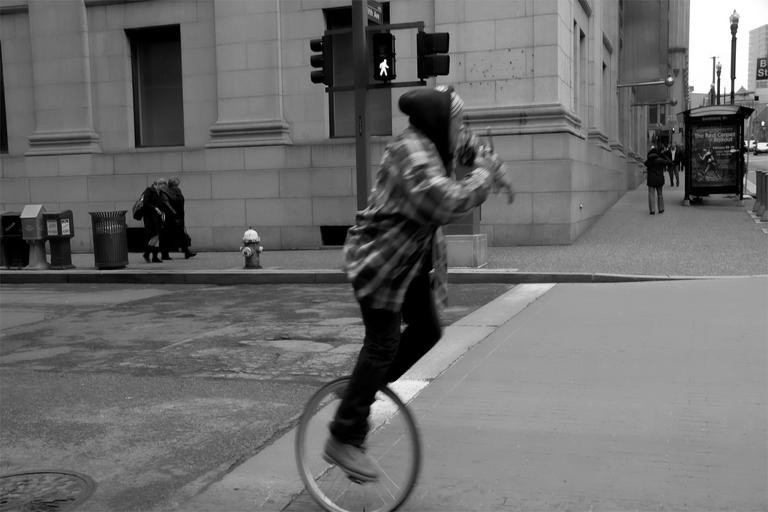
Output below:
[162,256,172,260]
[185,251,196,259]
[650,210,664,214]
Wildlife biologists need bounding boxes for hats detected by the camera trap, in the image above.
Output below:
[451,90,465,117]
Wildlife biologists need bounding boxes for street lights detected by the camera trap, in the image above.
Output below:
[710,83,715,105]
[729,9,740,106]
[716,61,723,104]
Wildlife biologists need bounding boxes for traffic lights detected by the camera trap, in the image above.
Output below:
[416,31,450,76]
[309,35,330,86]
[373,32,396,80]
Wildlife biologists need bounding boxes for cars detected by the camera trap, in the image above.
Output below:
[743,140,768,156]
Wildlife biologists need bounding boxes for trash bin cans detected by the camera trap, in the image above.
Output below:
[88,210,129,270]
[0,212,29,270]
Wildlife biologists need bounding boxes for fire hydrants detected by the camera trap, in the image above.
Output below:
[240,225,264,269]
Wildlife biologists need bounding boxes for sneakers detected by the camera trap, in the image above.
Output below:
[323,433,378,481]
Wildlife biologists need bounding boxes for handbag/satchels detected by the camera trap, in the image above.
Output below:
[133,199,144,220]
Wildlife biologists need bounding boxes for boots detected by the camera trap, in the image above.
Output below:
[143,245,162,263]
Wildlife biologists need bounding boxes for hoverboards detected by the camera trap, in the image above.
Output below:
[294,263,425,512]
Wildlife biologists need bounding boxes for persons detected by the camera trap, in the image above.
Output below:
[644,141,685,215]
[322,84,516,482]
[161,177,196,260]
[143,179,175,263]
